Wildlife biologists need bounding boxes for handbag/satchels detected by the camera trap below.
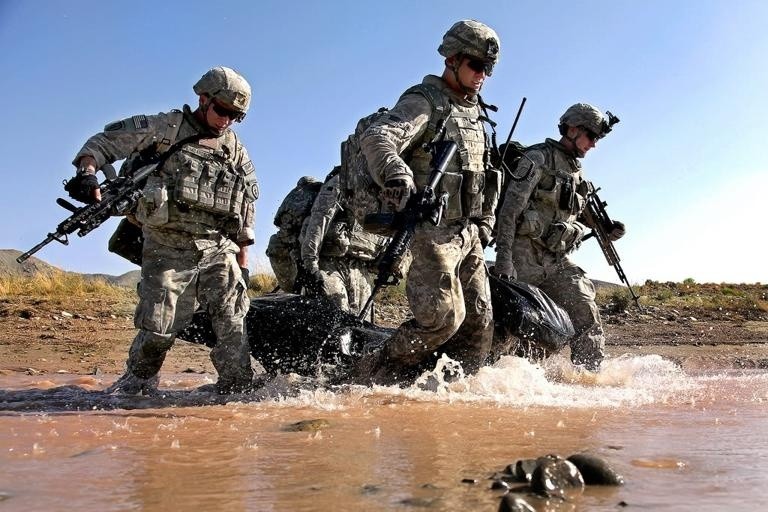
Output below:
[487,270,576,355]
[245,283,348,385]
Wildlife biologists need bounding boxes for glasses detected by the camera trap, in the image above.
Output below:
[203,93,246,124]
[452,53,494,77]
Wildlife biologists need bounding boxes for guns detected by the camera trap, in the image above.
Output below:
[353,139,459,327]
[16,149,164,266]
[574,176,644,314]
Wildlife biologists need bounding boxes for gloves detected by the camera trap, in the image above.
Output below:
[493,247,518,279]
[477,221,493,251]
[62,172,101,205]
[380,173,418,213]
[608,218,626,241]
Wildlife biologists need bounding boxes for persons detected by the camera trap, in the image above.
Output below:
[481,103,626,374]
[63,67,260,393]
[299,167,406,354]
[357,15,503,392]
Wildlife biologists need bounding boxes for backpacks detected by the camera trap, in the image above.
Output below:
[264,175,324,295]
[337,83,445,240]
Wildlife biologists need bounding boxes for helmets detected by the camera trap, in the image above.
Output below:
[437,18,501,65]
[192,65,252,114]
[558,102,608,139]
